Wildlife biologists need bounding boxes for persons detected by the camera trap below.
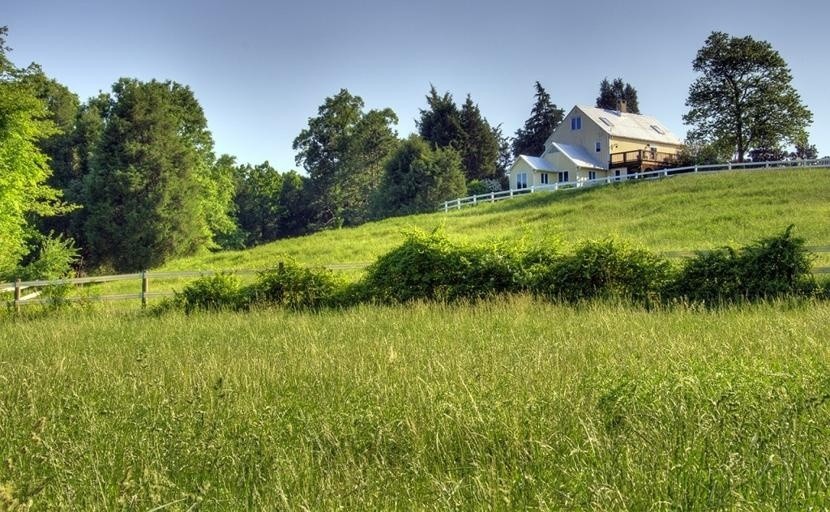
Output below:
[644,143,651,160]
